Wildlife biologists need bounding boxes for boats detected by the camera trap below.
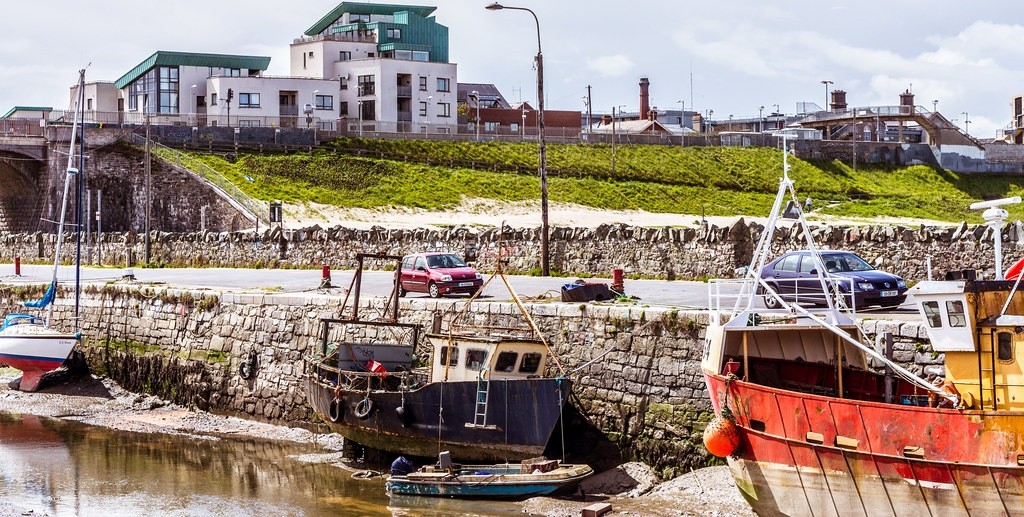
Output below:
[296,220,574,464]
[694,130,1023,517]
[386,380,596,498]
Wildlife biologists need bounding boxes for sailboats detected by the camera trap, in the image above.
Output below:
[0,60,94,396]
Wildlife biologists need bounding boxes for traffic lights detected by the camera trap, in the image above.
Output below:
[227,89,233,100]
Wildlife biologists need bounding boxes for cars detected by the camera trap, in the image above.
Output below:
[753,249,909,314]
[393,251,484,298]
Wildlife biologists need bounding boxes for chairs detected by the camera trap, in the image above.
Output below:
[24,279,57,308]
[780,362,837,396]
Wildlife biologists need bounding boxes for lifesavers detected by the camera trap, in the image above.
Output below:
[394,407,410,430]
[350,471,374,480]
[354,398,376,420]
[238,362,255,380]
[328,397,344,424]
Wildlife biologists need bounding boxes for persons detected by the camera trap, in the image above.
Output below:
[925,374,961,406]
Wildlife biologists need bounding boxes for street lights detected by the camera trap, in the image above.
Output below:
[618,104,627,143]
[758,105,765,133]
[355,84,364,137]
[582,96,589,140]
[521,100,530,141]
[484,1,551,277]
[773,103,780,129]
[729,114,734,131]
[310,90,319,145]
[705,108,714,141]
[820,81,835,112]
[189,84,197,125]
[471,90,480,142]
[676,100,685,146]
[960,111,971,134]
[425,95,433,138]
